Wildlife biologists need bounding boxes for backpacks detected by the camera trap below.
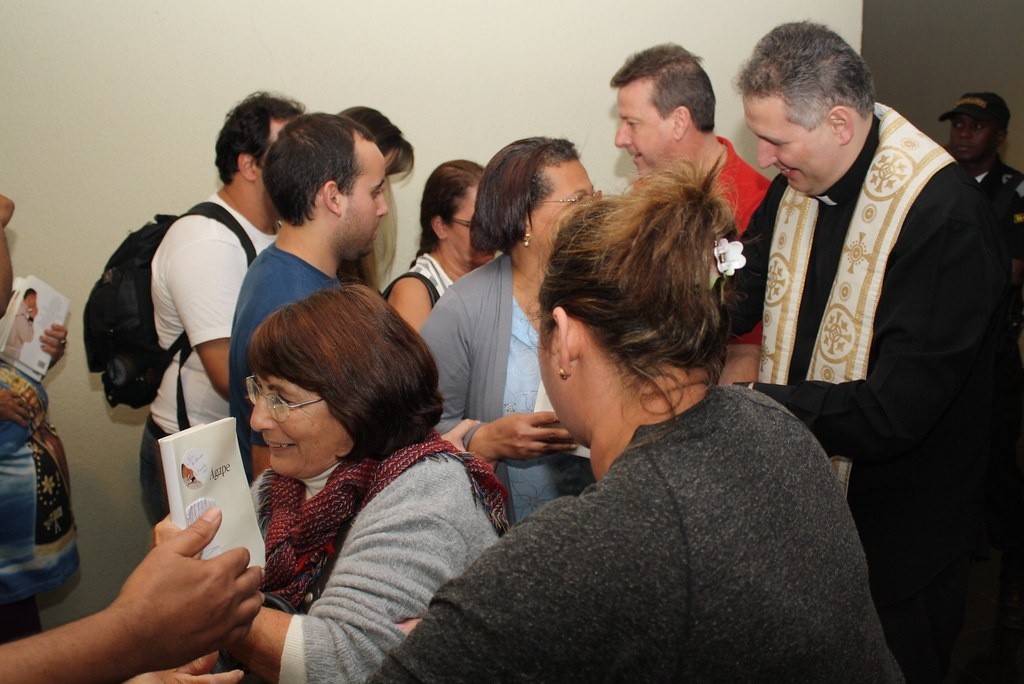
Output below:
[83,203,257,409]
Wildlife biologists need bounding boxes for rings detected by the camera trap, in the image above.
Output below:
[61,340,66,344]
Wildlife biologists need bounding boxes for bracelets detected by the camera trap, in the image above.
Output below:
[28,317,33,322]
[361,249,374,257]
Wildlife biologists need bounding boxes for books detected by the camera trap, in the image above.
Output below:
[0,274,70,384]
[158,416,268,569]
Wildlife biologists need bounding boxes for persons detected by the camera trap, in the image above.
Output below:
[227,105,497,490]
[0,505,266,684]
[611,45,774,387]
[138,97,307,523]
[0,195,81,646]
[152,283,510,684]
[379,159,499,338]
[365,160,910,684]
[712,22,1013,684]
[939,95,1023,633]
[419,136,604,544]
[4,287,38,359]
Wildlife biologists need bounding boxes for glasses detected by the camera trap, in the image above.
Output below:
[245,375,325,423]
[531,190,603,205]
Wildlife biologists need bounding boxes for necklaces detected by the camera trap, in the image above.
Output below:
[223,187,240,212]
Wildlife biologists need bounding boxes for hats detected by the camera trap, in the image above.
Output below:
[939,91,1009,129]
